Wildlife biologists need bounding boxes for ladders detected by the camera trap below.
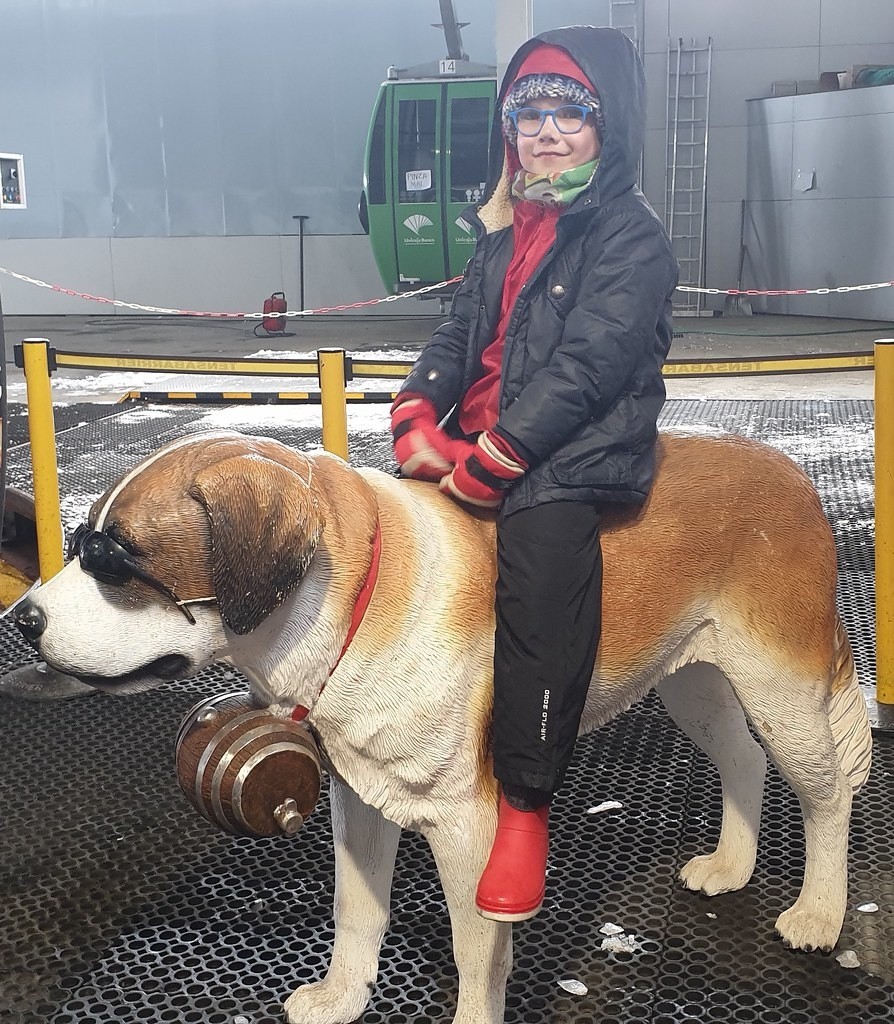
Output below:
[664,31,724,319]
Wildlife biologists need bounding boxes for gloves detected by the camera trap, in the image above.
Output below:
[438,430,526,510]
[391,399,471,482]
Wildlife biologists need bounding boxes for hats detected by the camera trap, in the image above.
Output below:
[502,74,605,152]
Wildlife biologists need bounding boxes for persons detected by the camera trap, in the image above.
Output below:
[390,25,680,923]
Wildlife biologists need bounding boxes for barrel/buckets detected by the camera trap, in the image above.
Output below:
[173,691,323,839]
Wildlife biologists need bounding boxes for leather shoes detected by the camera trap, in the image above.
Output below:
[474,794,550,922]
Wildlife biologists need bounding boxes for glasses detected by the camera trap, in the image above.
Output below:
[507,104,593,136]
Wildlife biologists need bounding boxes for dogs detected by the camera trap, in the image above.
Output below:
[10,429,876,1024]
